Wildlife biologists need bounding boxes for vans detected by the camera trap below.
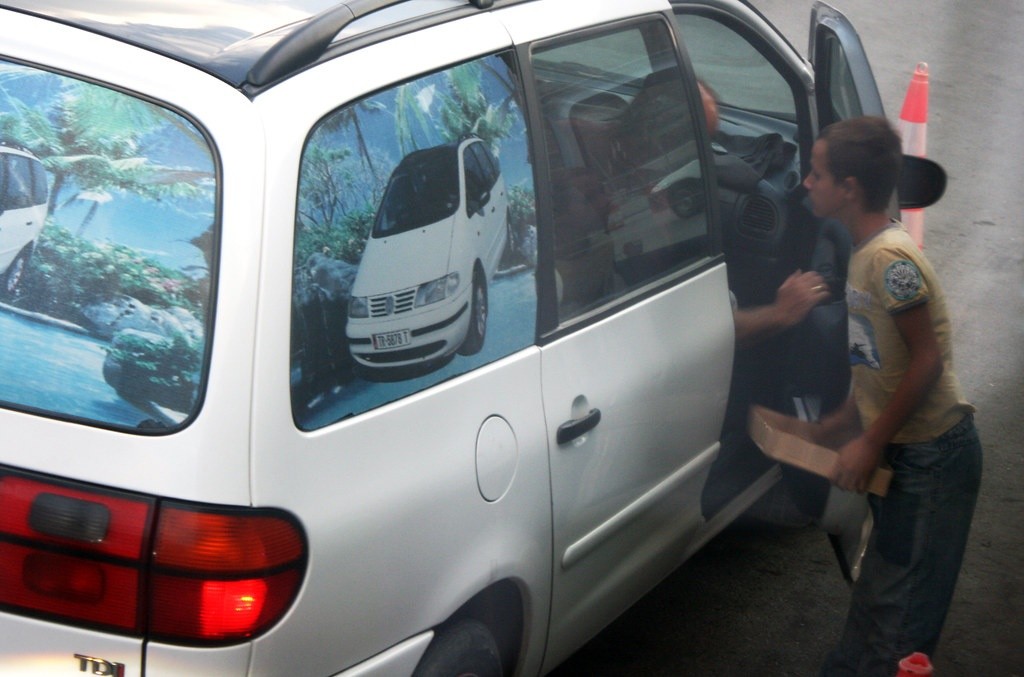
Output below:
[343,135,508,376]
[0,1,891,677]
[0,134,48,294]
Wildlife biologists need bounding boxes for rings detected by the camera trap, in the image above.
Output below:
[814,286,823,291]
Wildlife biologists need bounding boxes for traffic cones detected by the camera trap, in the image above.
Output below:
[891,57,930,253]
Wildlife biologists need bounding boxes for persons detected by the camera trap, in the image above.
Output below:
[557,70,831,430]
[804,116,982,677]
